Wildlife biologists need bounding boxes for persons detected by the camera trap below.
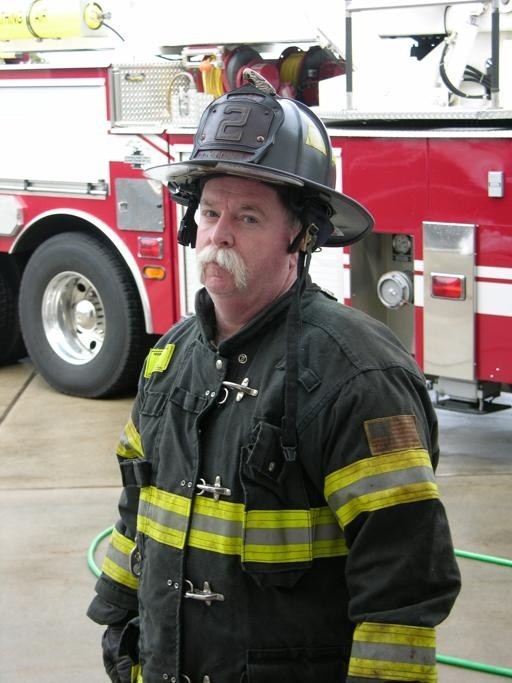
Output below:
[83,174,460,680]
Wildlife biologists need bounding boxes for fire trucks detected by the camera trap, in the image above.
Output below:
[2,0,512,415]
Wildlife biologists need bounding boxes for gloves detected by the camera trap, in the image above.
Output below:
[101,620,142,683]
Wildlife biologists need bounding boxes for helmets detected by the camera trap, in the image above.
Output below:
[143,85,383,251]
